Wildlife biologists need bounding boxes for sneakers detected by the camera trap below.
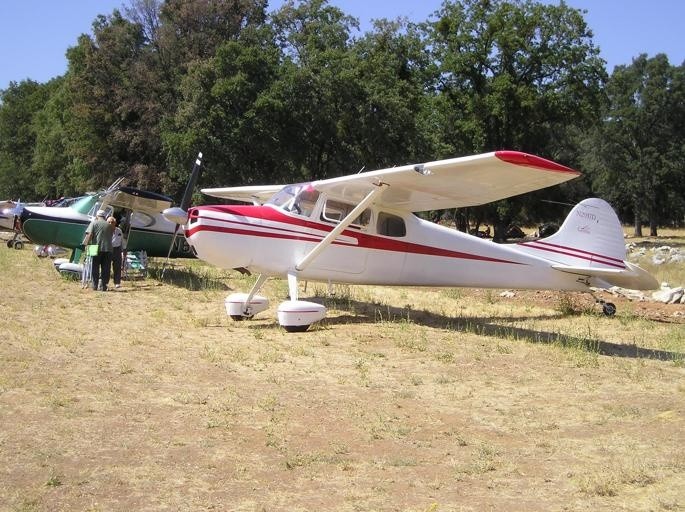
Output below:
[92,284,120,291]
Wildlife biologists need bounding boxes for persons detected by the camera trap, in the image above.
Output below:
[80,208,115,291]
[105,216,127,288]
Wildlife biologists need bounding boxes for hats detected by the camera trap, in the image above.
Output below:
[97,210,106,216]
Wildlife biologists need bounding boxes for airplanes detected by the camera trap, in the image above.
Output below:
[120,149,660,333]
[0,192,97,257]
[10,153,203,280]
